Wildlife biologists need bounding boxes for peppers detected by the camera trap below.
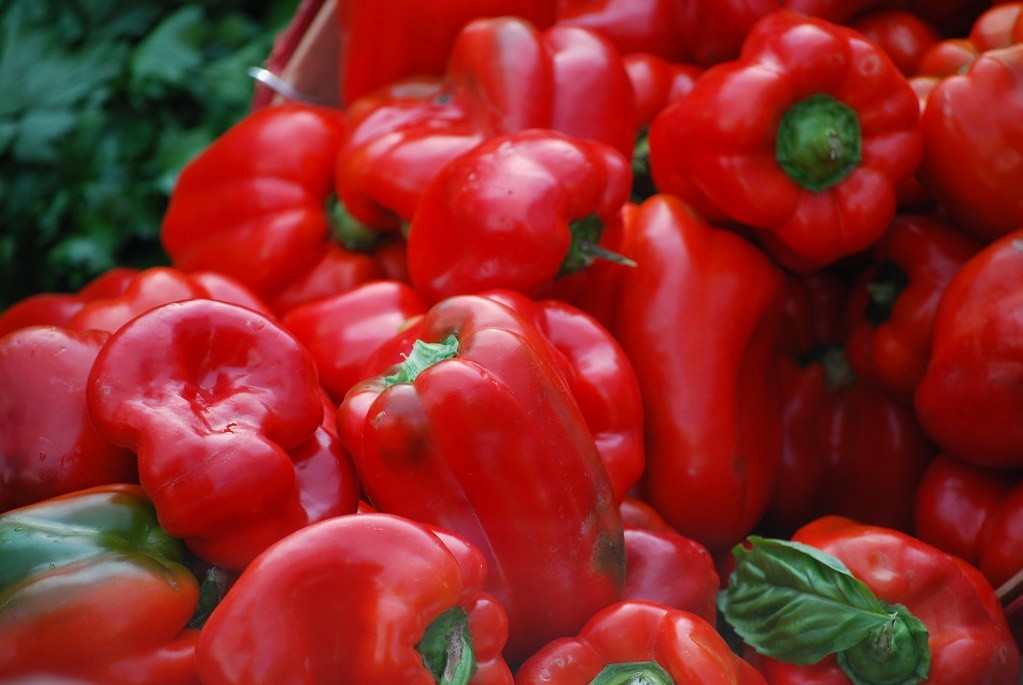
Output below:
[0,0,1023,685]
